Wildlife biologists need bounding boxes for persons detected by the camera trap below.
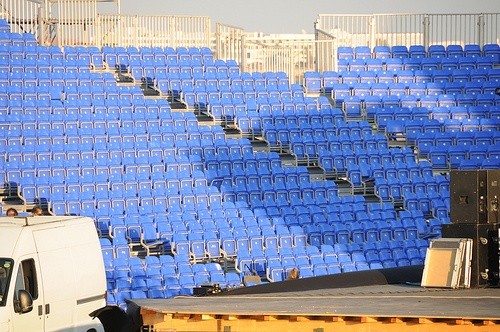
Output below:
[287,267,299,280]
[7,208,19,217]
[32,206,43,218]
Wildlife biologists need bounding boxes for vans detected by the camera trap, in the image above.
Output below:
[0,216,108,332]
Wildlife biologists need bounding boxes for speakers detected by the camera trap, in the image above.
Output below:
[441,169,500,289]
